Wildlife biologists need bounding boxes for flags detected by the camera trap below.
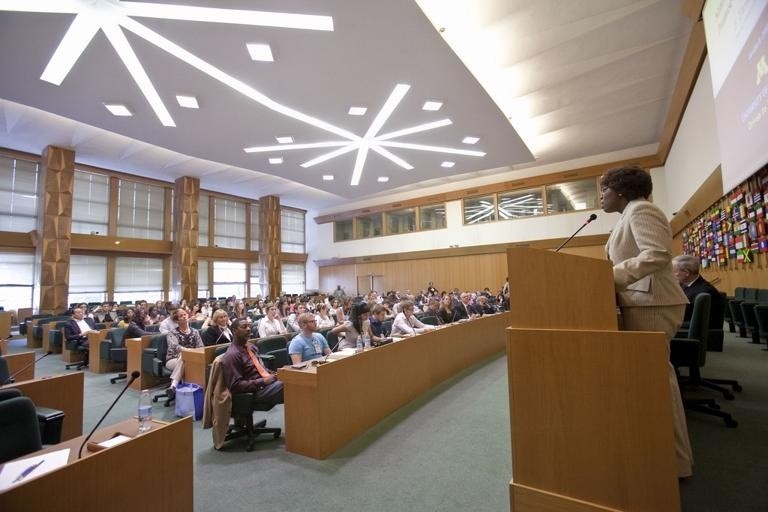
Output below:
[680,170,767,270]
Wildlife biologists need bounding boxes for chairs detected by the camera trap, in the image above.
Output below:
[669,288,744,430]
[724,286,768,352]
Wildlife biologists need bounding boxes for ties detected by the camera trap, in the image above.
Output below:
[243,344,271,379]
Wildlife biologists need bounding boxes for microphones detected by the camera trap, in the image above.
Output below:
[78,370,141,459]
[402,310,417,335]
[4,334,13,340]
[319,336,346,365]
[550,213,599,254]
[214,324,230,345]
[288,313,299,332]
[452,307,459,322]
[9,350,53,383]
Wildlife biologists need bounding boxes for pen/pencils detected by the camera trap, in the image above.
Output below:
[12,460,44,483]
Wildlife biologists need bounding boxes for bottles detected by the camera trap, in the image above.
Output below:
[138,389,153,431]
[356,336,363,351]
[365,332,370,349]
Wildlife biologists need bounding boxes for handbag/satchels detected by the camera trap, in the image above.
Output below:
[175,378,204,423]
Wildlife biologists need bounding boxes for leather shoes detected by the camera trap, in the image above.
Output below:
[166,388,176,400]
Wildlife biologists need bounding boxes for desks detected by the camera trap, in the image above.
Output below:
[0,349,194,509]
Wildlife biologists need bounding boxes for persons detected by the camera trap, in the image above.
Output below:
[599,166,695,482]
[164,309,204,397]
[502,277,510,298]
[64,307,98,367]
[288,312,333,366]
[670,254,721,322]
[70,288,509,352]
[427,282,438,296]
[332,285,346,299]
[221,317,285,405]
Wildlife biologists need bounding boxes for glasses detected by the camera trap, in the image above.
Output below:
[304,320,317,324]
[599,186,609,195]
